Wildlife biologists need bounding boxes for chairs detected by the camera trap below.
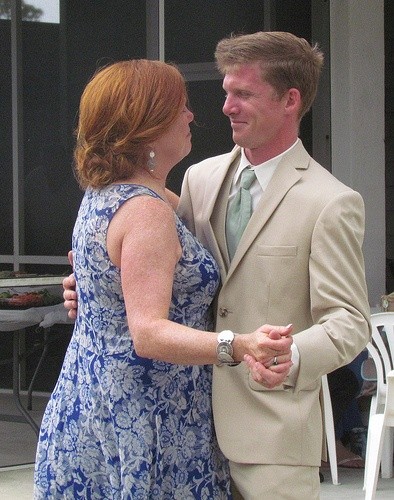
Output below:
[362,312,394,500]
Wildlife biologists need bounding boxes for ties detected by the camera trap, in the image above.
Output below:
[225,168,257,264]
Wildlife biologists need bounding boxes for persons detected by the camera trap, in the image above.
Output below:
[61,31,372,500]
[32,59,292,500]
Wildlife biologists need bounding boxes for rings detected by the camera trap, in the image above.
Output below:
[274,357,278,365]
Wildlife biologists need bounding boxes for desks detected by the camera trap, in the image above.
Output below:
[0,285,77,472]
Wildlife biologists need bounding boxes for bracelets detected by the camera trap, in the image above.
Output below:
[215,329,242,368]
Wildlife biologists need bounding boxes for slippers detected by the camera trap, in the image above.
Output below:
[327,454,363,472]
